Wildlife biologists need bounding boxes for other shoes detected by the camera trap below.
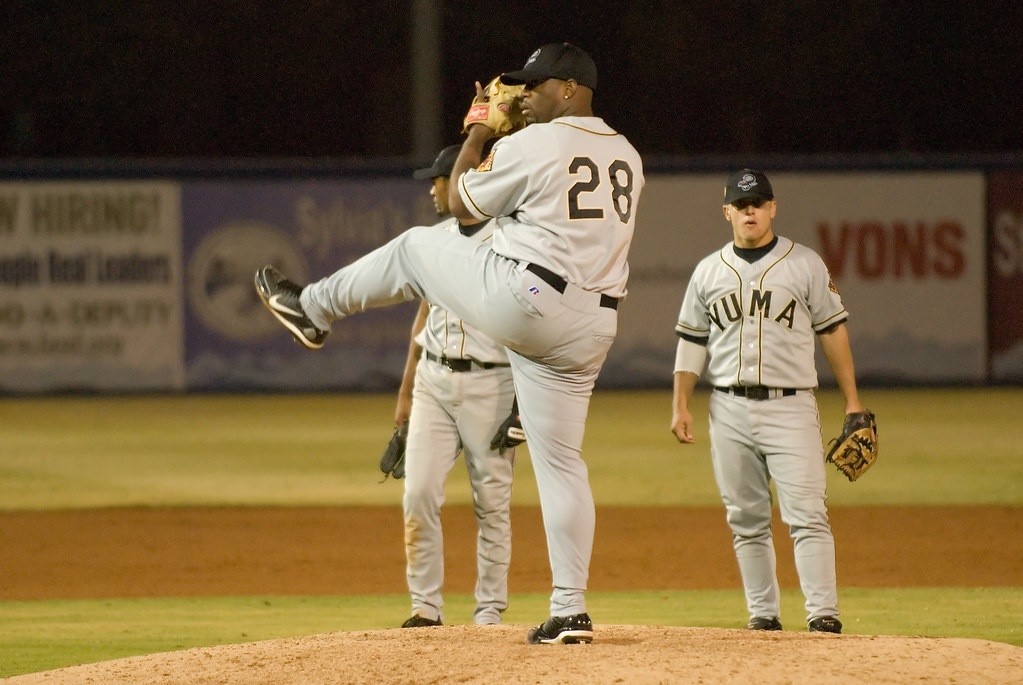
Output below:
[402,615,442,628]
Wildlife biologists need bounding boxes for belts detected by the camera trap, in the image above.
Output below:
[526,264,618,311]
[716,386,796,401]
[427,351,511,372]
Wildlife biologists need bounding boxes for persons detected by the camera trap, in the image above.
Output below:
[670,167,862,635]
[381,146,526,628]
[252,43,646,644]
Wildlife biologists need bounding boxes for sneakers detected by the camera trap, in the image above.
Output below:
[748,617,782,630]
[254,265,329,350]
[527,613,593,645]
[807,616,842,633]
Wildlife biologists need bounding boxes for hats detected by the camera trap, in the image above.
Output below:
[500,43,598,92]
[413,145,486,181]
[723,168,773,204]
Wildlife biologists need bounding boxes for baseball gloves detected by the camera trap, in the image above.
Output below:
[459,73,527,138]
[823,408,882,483]
[375,419,409,485]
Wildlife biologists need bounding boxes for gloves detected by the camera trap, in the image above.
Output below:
[490,412,526,455]
